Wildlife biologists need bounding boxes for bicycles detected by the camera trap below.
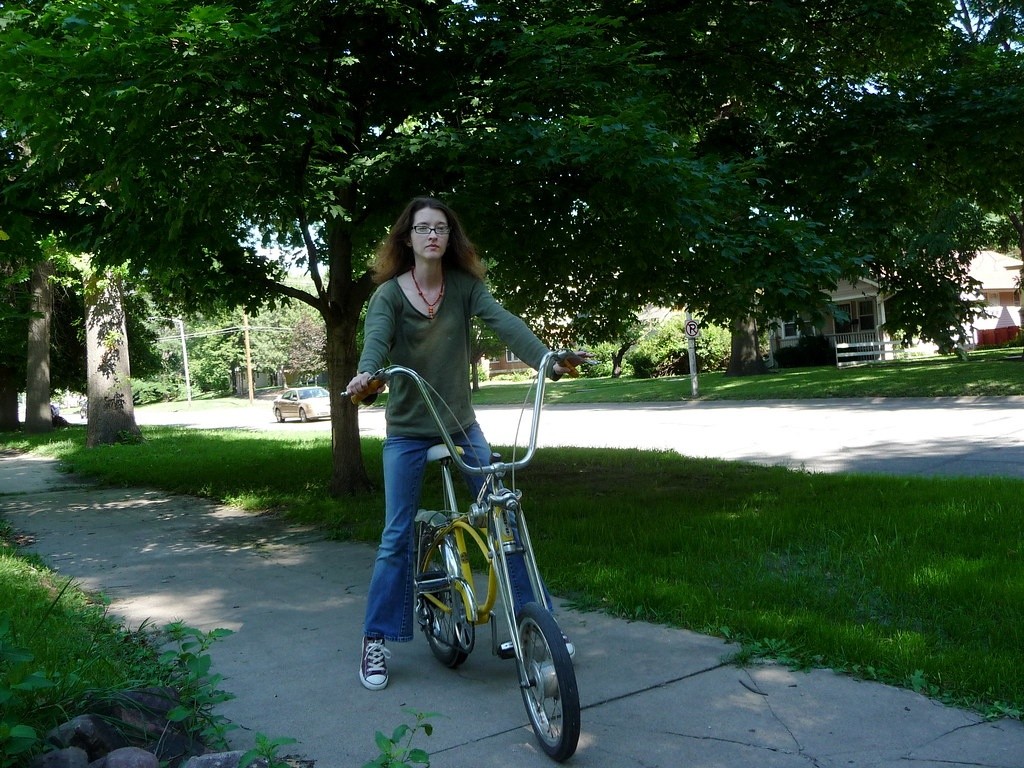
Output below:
[341,348,600,763]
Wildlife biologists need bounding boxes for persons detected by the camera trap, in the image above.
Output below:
[346,196,595,691]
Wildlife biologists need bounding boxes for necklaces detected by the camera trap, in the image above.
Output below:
[411,266,444,318]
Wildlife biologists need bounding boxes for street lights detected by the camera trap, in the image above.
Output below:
[145,316,193,406]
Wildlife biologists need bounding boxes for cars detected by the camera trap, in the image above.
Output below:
[272,387,331,423]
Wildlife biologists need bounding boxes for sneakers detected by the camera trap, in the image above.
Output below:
[359,635,393,690]
[546,631,576,661]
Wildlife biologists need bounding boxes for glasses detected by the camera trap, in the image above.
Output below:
[409,225,452,234]
